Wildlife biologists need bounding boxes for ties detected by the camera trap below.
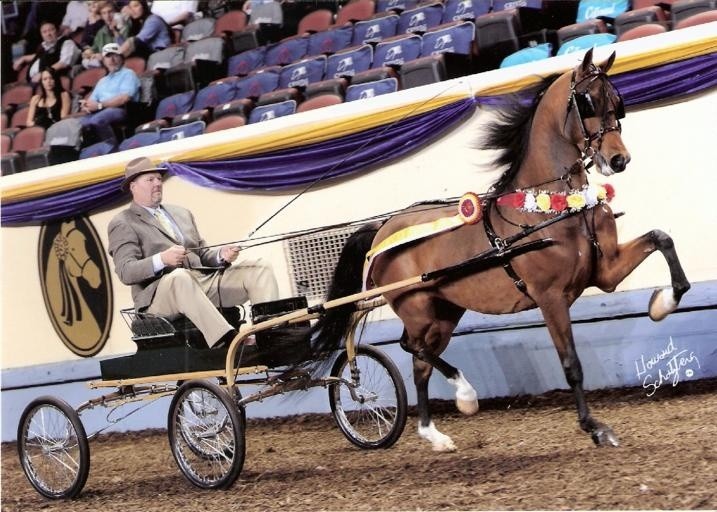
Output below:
[154,211,176,244]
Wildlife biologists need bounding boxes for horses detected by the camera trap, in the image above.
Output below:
[253,46,691,453]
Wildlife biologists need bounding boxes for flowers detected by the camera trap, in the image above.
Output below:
[507,180,617,218]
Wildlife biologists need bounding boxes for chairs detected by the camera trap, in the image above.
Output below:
[2,0,717,175]
[117,303,246,343]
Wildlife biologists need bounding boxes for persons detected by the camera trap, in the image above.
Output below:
[12,1,283,129]
[106,157,279,349]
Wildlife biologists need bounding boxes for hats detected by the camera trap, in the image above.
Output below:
[120,155,170,194]
[101,42,124,58]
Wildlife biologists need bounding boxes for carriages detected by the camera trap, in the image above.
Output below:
[16,46,690,498]
